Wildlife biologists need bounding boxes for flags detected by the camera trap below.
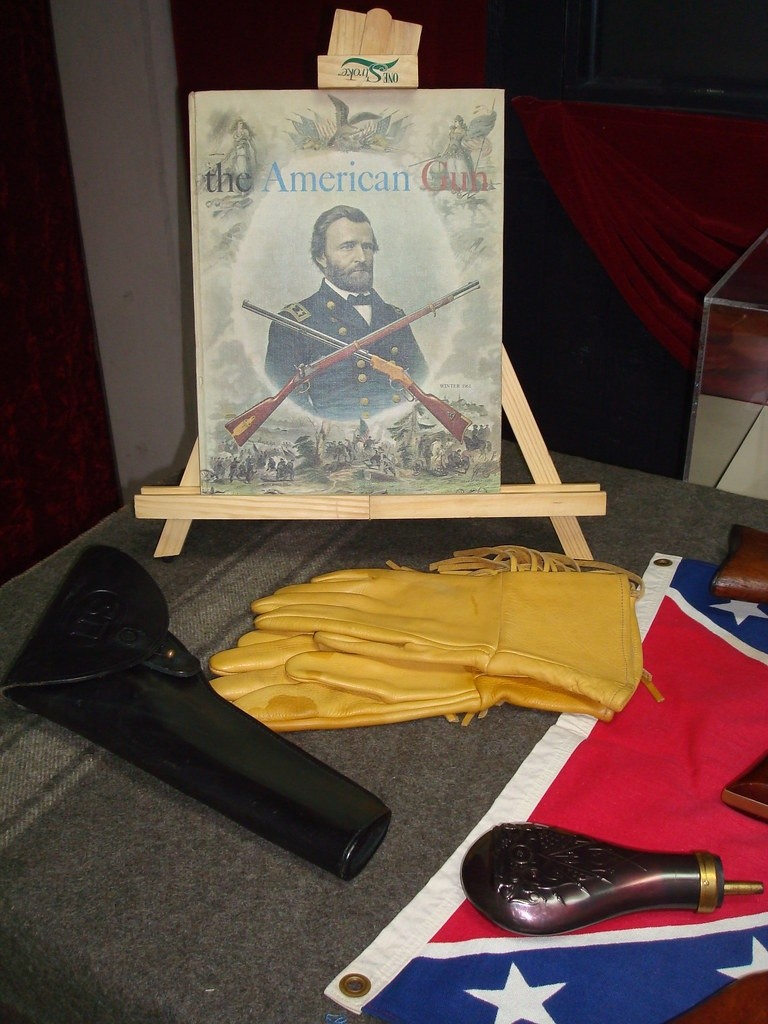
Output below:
[324,552,768,1024]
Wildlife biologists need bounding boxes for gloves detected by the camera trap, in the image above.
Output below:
[207,545,663,723]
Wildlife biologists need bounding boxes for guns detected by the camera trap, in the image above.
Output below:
[709,524,768,604]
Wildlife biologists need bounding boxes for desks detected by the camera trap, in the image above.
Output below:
[0,436,768,1024]
